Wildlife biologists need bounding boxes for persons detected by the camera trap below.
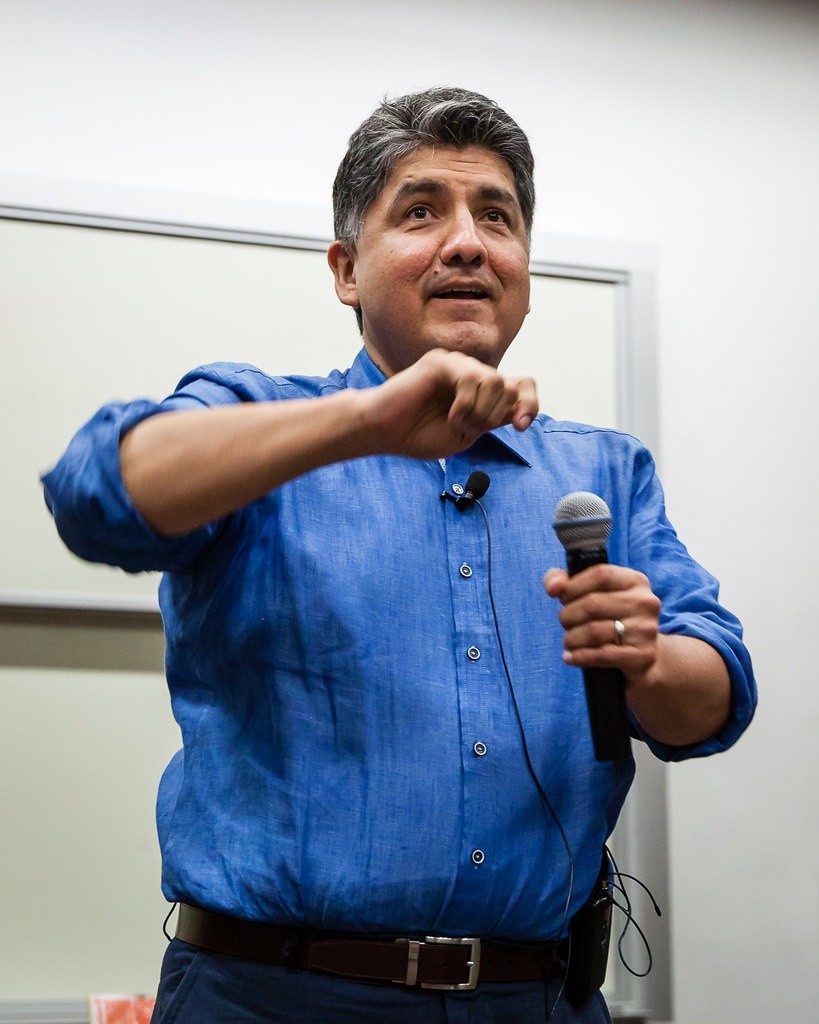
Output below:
[41,89,759,1024]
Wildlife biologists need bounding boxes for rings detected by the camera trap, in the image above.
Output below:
[613,618,625,645]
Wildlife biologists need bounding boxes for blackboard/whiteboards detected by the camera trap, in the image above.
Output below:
[3,203,631,623]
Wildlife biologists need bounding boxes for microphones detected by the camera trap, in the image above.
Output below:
[551,491,632,764]
[456,471,490,514]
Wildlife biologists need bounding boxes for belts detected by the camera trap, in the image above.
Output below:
[174,902,573,991]
